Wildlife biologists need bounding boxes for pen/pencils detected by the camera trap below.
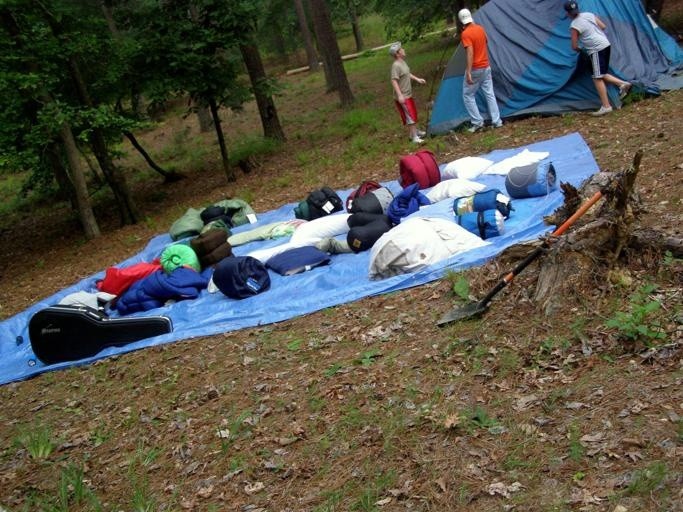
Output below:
[434,166,627,327]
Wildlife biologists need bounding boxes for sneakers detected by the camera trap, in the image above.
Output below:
[618,81,631,99]
[592,106,614,117]
[467,119,502,132]
[407,131,426,145]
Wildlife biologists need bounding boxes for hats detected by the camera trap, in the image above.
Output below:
[565,1,578,15]
[457,9,472,26]
[389,42,400,56]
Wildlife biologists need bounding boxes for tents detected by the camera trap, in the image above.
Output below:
[424,0,683,138]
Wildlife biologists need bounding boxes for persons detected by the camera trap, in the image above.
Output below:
[388,40,429,146]
[562,1,633,118]
[457,6,506,134]
